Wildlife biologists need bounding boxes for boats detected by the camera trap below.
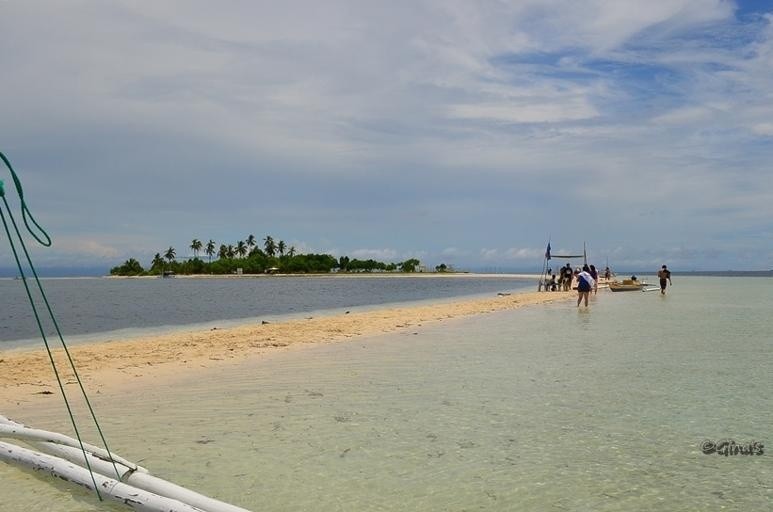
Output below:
[607,277,645,292]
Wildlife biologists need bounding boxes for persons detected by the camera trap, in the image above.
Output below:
[605,267,610,280]
[658,264,672,295]
[631,274,637,280]
[544,263,599,307]
